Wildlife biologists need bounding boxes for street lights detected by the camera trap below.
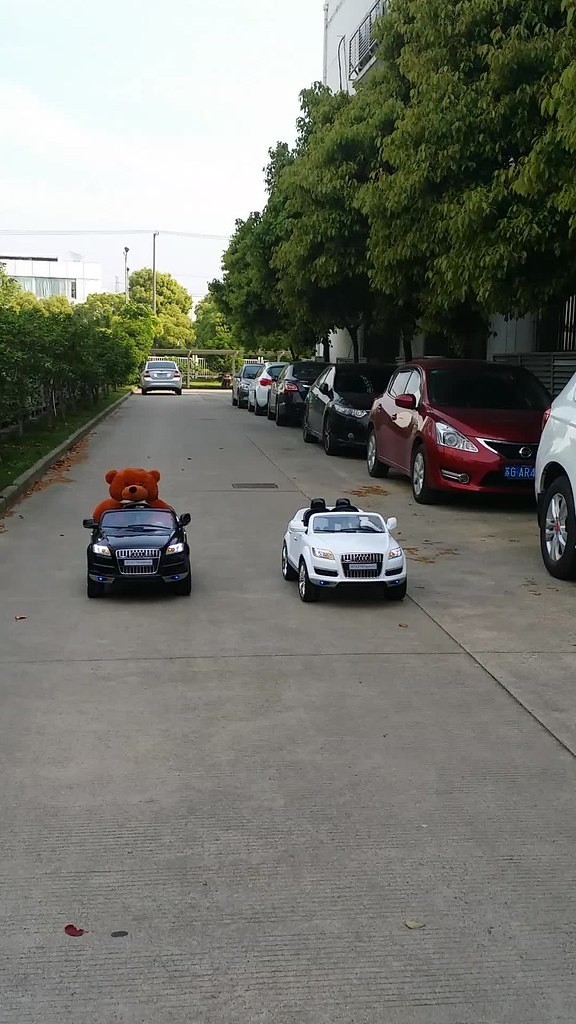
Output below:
[152,230,160,312]
[123,246,130,304]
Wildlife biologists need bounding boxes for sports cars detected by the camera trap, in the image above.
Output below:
[82,502,192,599]
[280,497,407,602]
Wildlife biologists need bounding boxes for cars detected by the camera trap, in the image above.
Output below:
[303,362,410,456]
[533,371,576,582]
[365,355,556,505]
[247,361,292,416]
[139,360,184,395]
[272,360,335,427]
[232,364,264,409]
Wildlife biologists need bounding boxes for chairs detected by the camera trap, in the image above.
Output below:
[303,498,331,528]
[330,497,360,514]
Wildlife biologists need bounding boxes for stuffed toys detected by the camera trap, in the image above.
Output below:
[92,468,173,523]
